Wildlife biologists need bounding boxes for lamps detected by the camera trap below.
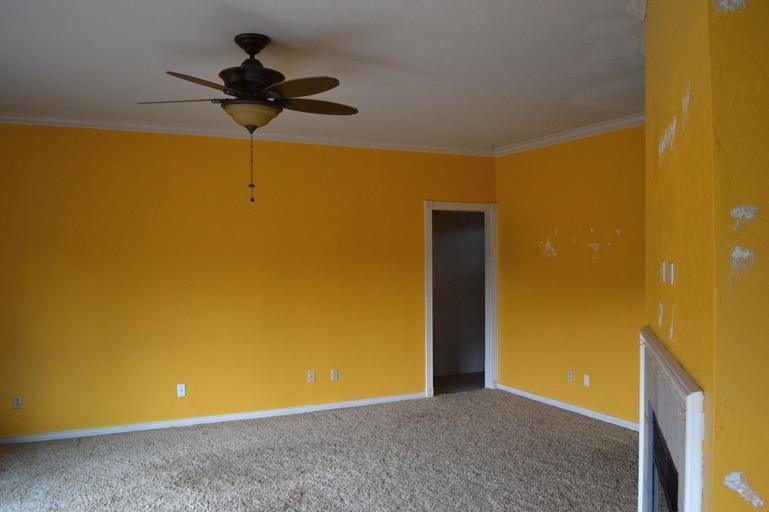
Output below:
[219,91,281,133]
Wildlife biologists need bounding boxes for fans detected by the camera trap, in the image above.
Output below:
[135,33,362,117]
